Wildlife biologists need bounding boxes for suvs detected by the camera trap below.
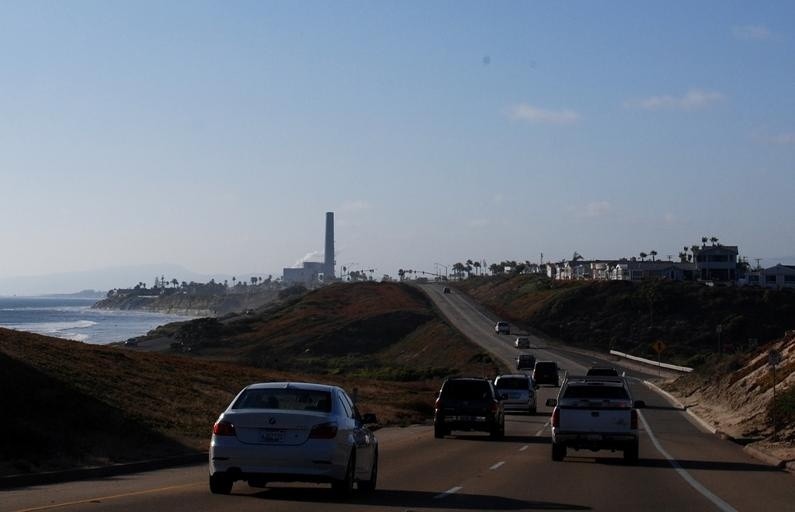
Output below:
[544,371,647,465]
[494,321,511,335]
[513,336,530,349]
[443,287,450,294]
[585,365,620,377]
[514,353,536,370]
[432,376,506,441]
[492,373,539,414]
[531,360,560,386]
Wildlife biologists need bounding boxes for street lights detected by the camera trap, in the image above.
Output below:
[433,262,448,281]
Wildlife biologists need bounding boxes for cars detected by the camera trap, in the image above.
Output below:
[123,338,138,347]
[246,308,256,315]
[207,379,381,501]
